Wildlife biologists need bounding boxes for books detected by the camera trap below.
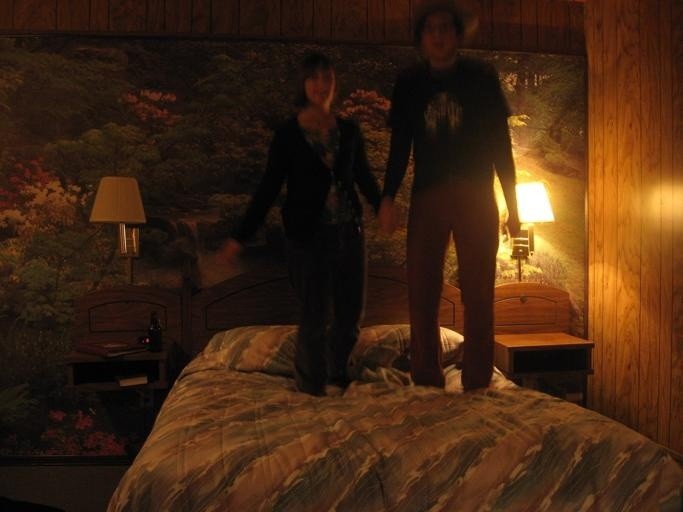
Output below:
[75,341,147,359]
[116,373,148,388]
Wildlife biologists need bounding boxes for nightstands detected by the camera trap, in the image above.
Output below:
[64,287,183,393]
[493,282,595,379]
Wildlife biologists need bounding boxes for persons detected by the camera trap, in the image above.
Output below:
[377,6,518,393]
[216,53,382,397]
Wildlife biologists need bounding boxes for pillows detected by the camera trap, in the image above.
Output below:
[199,321,466,379]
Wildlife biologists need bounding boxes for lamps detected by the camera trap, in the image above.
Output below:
[89,175,147,258]
[510,181,554,260]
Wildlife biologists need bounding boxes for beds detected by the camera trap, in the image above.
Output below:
[104,263,683,512]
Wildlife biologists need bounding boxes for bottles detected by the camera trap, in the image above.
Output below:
[148,311,163,353]
